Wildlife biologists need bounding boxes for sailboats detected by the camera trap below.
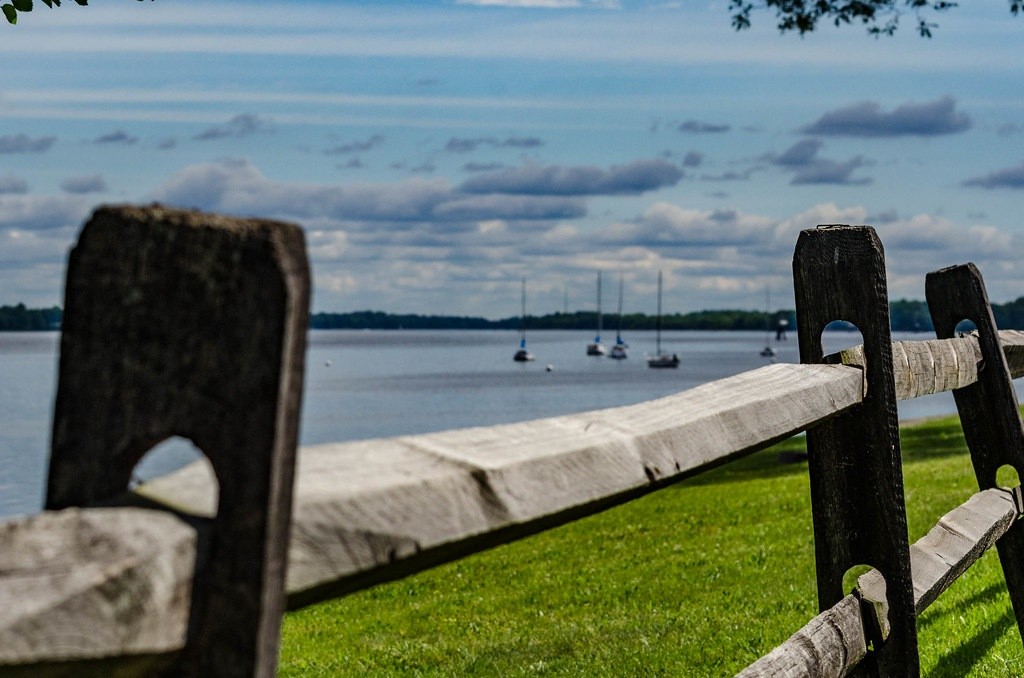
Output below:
[645,271,679,369]
[610,275,629,358]
[586,269,605,357]
[760,283,774,357]
[512,277,534,362]
